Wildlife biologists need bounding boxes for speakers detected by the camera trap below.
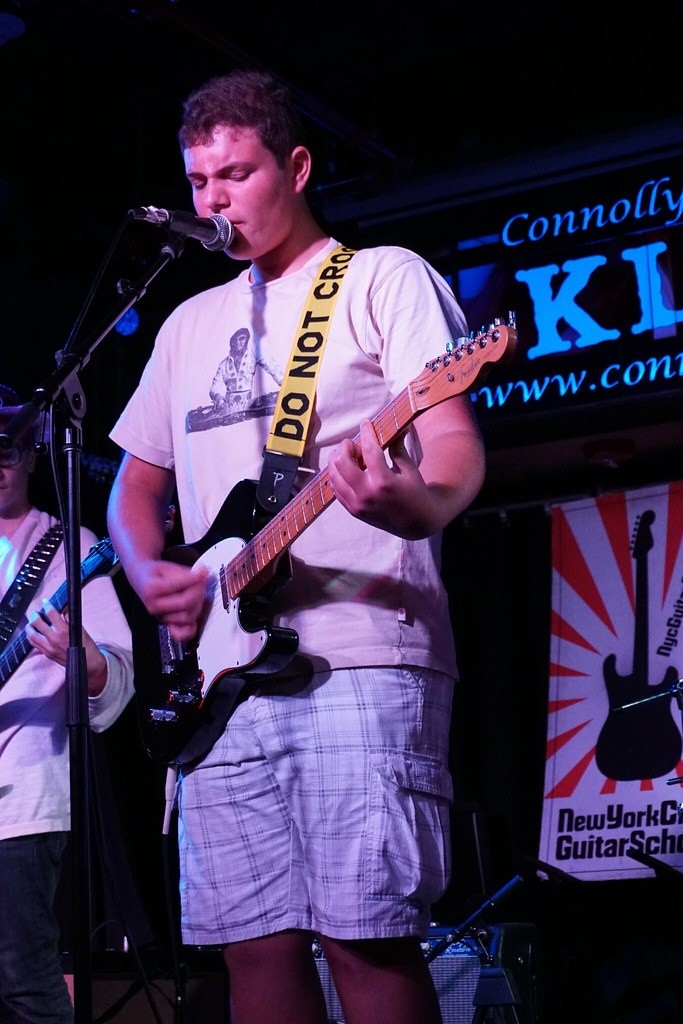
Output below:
[312,922,535,1024]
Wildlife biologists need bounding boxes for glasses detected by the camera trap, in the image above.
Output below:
[0,445,32,467]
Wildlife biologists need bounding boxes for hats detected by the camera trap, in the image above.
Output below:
[0,382,21,422]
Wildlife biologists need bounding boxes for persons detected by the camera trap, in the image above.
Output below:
[0,386,133,1024]
[108,77,487,1024]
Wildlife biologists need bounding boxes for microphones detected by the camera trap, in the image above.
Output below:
[131,205,234,251]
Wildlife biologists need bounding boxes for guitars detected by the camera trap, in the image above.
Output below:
[0,504,177,693]
[129,309,519,767]
